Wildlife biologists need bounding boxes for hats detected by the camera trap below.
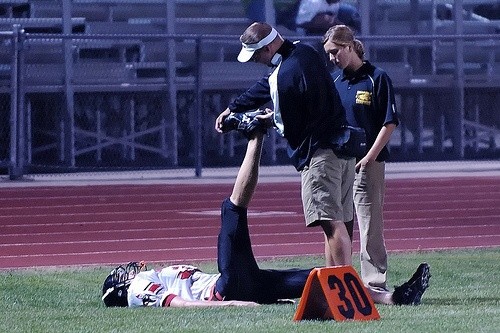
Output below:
[236,26,278,63]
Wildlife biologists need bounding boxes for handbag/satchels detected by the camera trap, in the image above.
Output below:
[331,126,367,159]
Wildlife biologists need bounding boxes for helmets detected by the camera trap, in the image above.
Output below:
[103,266,135,307]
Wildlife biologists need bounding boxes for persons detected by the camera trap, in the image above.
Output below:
[321,24,400,291]
[101,111,431,307]
[215,22,357,268]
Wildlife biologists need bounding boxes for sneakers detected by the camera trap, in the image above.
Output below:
[392,262,431,305]
[219,108,274,138]
